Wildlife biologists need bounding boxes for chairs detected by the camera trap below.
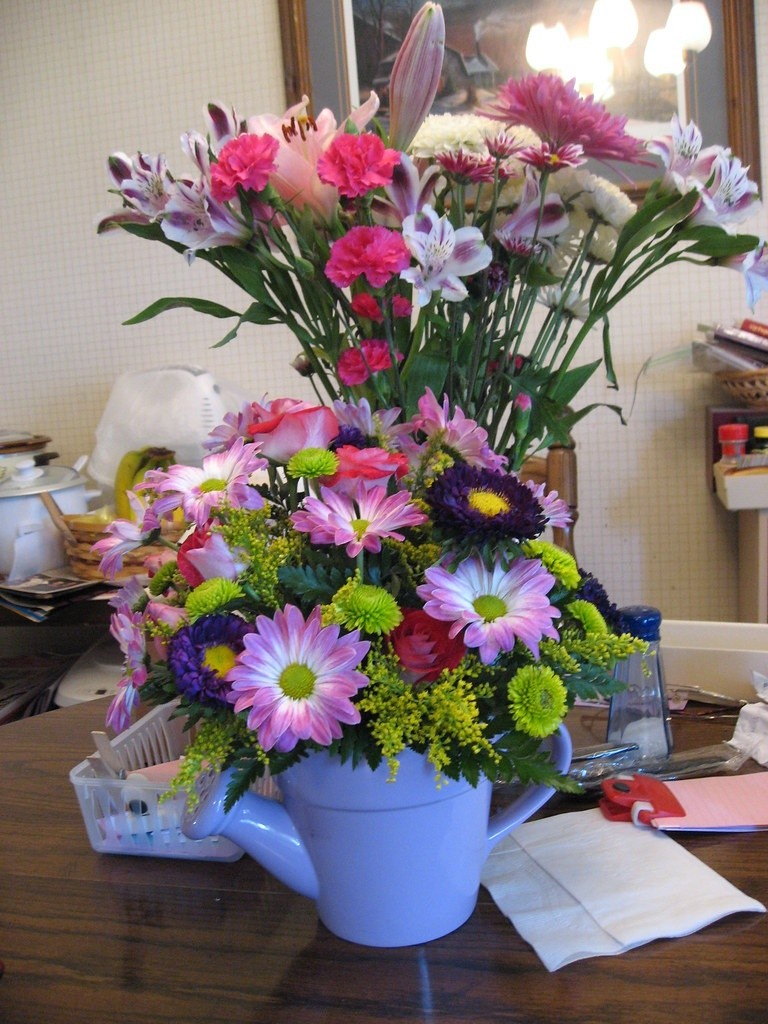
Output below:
[510,407,579,567]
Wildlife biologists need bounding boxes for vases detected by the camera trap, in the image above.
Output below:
[178,714,572,946]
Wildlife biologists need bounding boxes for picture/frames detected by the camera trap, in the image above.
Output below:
[277,0,762,229]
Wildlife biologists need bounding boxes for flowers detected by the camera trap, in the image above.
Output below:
[95,386,658,826]
[96,0,768,476]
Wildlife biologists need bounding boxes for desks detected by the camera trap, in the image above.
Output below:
[0,702,768,1024]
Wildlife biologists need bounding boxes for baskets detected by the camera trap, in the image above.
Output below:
[713,369,768,409]
[69,695,285,861]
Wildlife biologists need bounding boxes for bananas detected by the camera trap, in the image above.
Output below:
[114,448,183,525]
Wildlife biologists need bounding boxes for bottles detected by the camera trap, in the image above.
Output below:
[748,425,768,456]
[604,606,675,759]
[712,423,750,484]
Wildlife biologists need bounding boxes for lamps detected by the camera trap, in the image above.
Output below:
[523,2,711,101]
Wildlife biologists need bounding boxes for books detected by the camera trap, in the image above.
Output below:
[0,564,108,623]
[691,318,768,370]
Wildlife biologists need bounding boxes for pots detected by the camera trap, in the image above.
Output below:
[0,429,103,578]
